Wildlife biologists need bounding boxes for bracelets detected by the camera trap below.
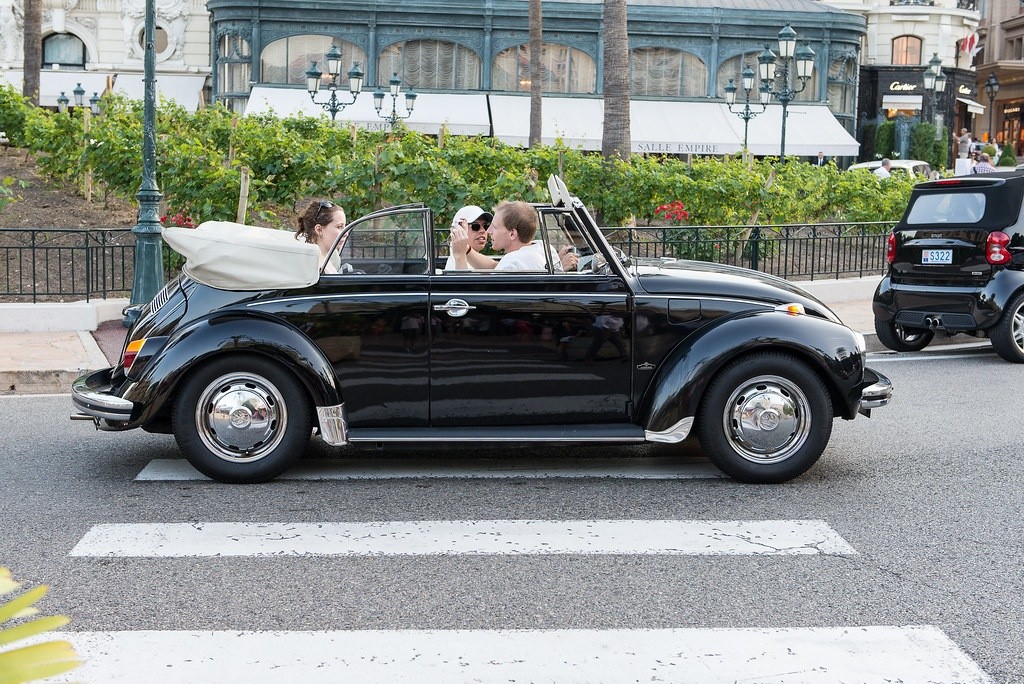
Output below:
[466,245,471,254]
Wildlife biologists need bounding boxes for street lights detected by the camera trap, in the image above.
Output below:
[985,72,998,142]
[758,22,815,164]
[725,66,772,152]
[922,53,947,124]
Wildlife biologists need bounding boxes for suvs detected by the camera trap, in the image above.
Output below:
[848,159,936,183]
[873,165,1024,364]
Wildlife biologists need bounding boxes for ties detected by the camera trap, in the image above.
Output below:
[820,159,822,166]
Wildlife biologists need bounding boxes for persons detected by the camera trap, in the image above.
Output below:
[873,158,891,181]
[294,199,349,274]
[812,152,828,167]
[952,127,999,175]
[443,204,579,272]
[450,201,565,272]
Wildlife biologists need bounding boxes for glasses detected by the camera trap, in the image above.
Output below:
[469,224,490,231]
[315,200,336,219]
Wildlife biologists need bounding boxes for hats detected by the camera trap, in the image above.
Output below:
[960,128,968,132]
[453,205,493,224]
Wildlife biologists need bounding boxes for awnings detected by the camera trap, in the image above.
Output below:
[111,74,206,121]
[956,97,986,115]
[0,71,115,107]
[488,94,861,156]
[241,86,491,137]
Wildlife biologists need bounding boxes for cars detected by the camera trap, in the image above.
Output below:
[69,175,893,484]
